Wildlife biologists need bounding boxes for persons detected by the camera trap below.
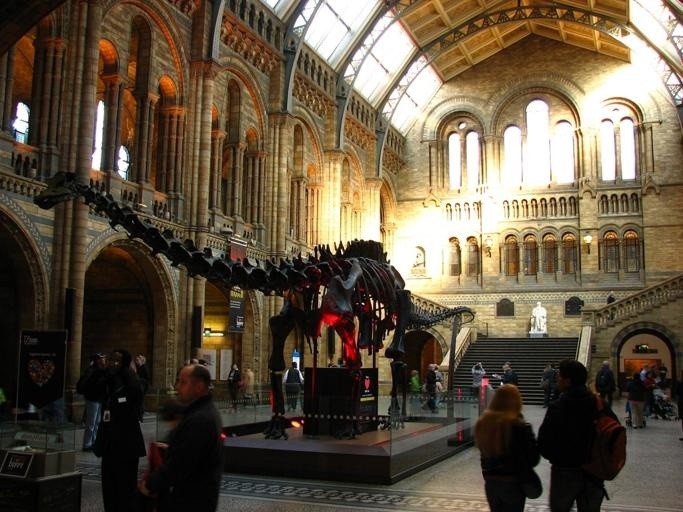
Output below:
[227,362,239,407]
[421,364,437,413]
[530,302,546,331]
[492,361,513,386]
[432,363,444,392]
[191,358,198,365]
[198,358,207,366]
[470,362,485,396]
[409,369,423,404]
[182,359,190,367]
[536,359,626,512]
[76,348,147,512]
[501,364,518,386]
[593,361,616,406]
[471,384,542,512]
[626,363,673,428]
[281,361,304,412]
[240,363,254,399]
[136,364,224,512]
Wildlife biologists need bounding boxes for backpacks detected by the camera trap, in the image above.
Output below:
[581,395,627,480]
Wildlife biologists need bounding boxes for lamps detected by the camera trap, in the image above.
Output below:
[484,236,493,257]
[584,232,592,255]
[203,331,224,337]
[220,228,233,235]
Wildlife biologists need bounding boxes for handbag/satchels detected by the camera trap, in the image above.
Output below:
[518,456,543,499]
